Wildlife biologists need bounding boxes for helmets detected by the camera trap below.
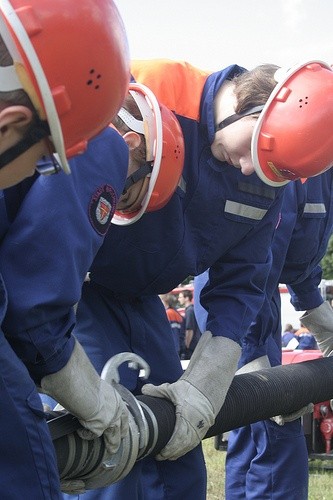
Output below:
[109,82,184,228]
[250,57,332,189]
[0,0,131,175]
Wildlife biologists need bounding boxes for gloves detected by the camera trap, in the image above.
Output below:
[298,300,332,359]
[233,355,315,426]
[34,336,131,455]
[140,328,243,463]
[57,479,87,497]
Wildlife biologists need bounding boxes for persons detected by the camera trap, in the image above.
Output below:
[193,166,333,500]
[38,58,333,500]
[0,83,185,500]
[179,290,202,359]
[157,294,183,359]
[281,321,319,350]
[0,0,130,500]
